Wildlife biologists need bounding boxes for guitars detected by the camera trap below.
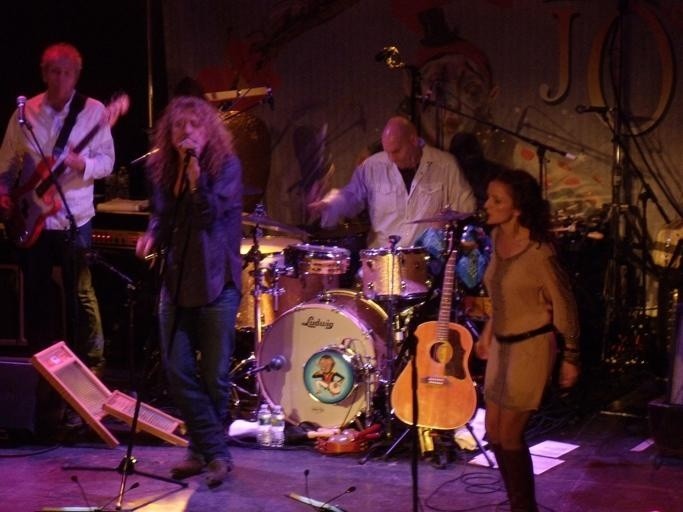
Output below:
[391,217,478,431]
[5,94,130,249]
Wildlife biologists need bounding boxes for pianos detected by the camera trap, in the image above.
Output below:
[94,229,301,253]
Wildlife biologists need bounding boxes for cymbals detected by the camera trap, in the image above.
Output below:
[402,210,473,225]
[240,211,307,236]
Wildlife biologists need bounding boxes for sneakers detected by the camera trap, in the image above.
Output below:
[170,453,235,485]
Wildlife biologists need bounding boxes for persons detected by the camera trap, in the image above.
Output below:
[0,43,116,428]
[474,167,582,511]
[135,95,243,486]
[307,116,477,251]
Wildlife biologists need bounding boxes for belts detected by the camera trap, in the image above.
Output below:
[494,323,554,344]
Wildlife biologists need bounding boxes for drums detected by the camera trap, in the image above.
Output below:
[359,247,434,303]
[287,244,351,275]
[234,236,344,332]
[256,289,401,433]
[463,290,491,320]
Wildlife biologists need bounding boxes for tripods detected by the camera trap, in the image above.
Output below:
[231,228,263,420]
[382,292,495,469]
[359,239,411,463]
[62,165,189,510]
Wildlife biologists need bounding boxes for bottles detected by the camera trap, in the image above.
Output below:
[256,404,271,446]
[270,404,285,445]
[228,418,381,445]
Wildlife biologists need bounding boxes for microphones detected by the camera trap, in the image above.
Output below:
[17,95,26,124]
[267,82,275,111]
[576,104,609,114]
[404,210,488,224]
[187,147,196,159]
